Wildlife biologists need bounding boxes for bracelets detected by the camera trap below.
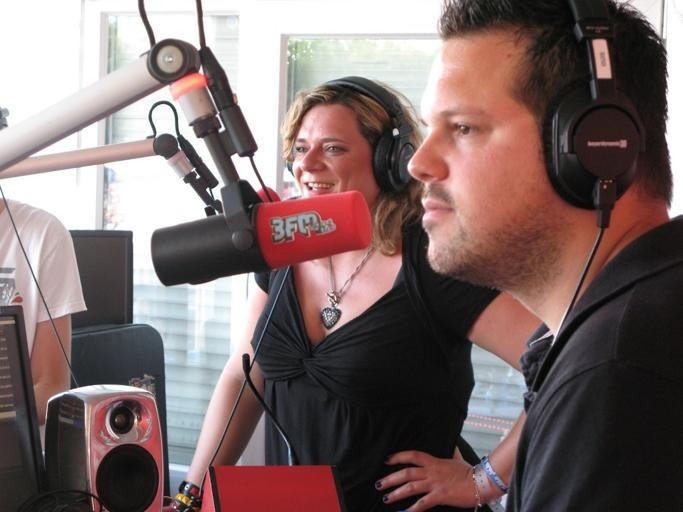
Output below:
[470,465,485,510]
[472,462,505,511]
[171,479,204,512]
[480,455,510,493]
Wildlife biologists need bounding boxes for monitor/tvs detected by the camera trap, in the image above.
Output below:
[0,305,45,512]
[69,229,133,328]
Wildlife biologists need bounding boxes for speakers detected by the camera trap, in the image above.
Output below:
[45,384,164,511]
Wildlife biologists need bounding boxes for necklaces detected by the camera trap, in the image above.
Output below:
[319,243,380,331]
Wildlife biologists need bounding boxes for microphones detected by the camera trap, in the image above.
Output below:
[150,190,372,286]
[242,354,301,466]
[257,187,281,203]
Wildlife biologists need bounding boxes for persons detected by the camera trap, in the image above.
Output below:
[0,106,88,457]
[160,74,557,512]
[403,0,681,512]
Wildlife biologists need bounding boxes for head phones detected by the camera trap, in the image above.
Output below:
[286,77,417,193]
[542,0,646,229]
